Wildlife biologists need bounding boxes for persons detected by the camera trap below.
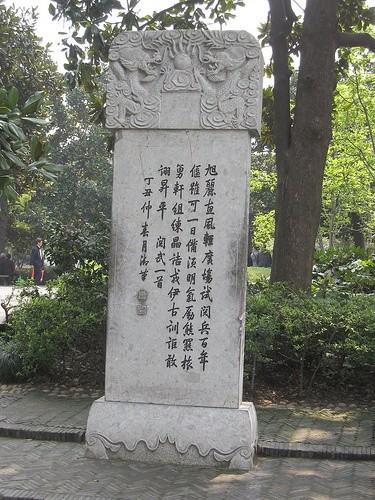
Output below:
[0,252,10,286]
[255,244,271,268]
[30,237,43,290]
[0,254,19,286]
[250,245,260,267]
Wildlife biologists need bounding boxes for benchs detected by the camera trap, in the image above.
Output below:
[0,275,10,285]
